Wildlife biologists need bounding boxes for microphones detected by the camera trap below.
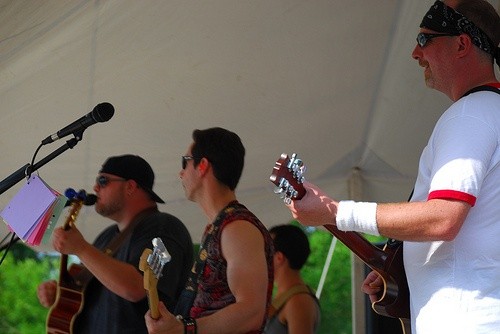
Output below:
[65,194,98,207]
[41,102,115,145]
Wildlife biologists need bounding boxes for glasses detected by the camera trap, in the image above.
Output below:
[416,32,474,48]
[95,175,130,188]
[181,156,204,170]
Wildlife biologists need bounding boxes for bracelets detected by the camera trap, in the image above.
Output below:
[182,317,198,334]
[335,199,380,237]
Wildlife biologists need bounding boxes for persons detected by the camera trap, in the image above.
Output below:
[38,155,193,334]
[144,127,275,333]
[267,223,321,334]
[285,1,500,334]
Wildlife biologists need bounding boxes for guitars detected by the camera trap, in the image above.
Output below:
[44,187,99,334]
[139,235,172,321]
[269,152,412,334]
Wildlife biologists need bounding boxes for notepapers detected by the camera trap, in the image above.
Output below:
[0,171,67,246]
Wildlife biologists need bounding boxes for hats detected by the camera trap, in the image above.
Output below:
[99,154,166,204]
